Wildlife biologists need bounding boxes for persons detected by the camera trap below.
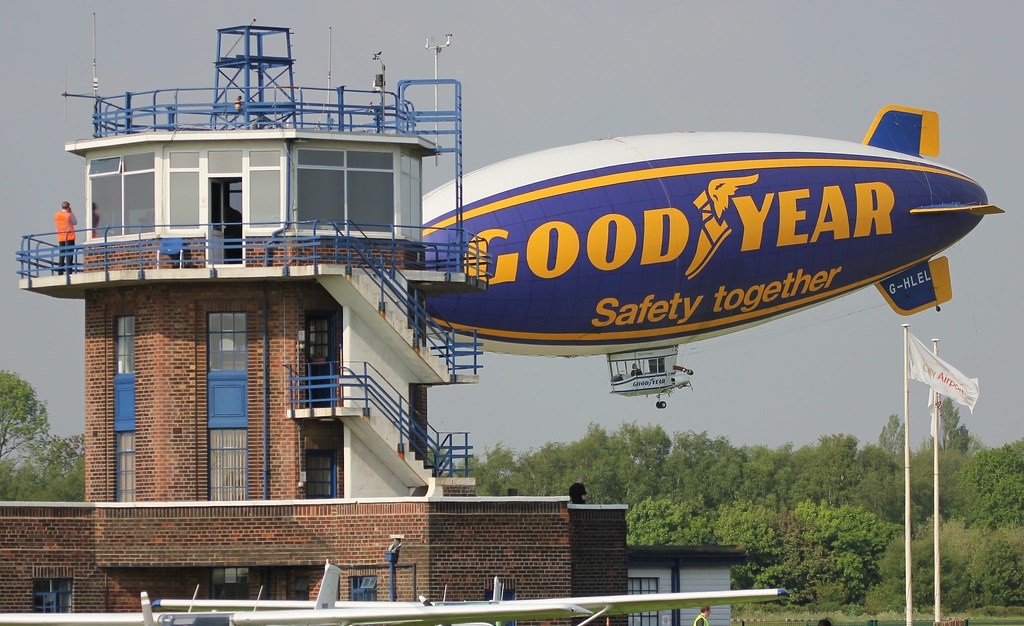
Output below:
[224,201,241,258]
[631,364,643,376]
[569,477,588,504]
[92,202,99,238]
[54,201,78,276]
[693,605,711,626]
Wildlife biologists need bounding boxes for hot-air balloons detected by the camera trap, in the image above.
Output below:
[421,104,1006,409]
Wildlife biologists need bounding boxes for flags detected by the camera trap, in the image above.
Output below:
[928,388,944,449]
[907,330,979,415]
